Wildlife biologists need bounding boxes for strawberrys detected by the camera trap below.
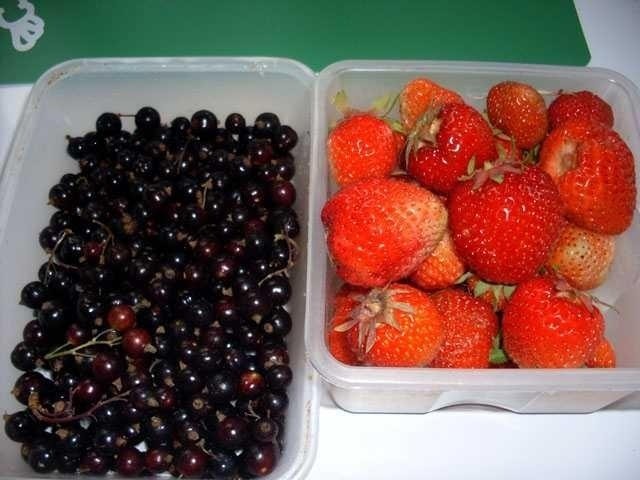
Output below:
[320,77,636,369]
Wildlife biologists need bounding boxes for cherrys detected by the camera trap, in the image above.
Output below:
[5,106,299,480]
